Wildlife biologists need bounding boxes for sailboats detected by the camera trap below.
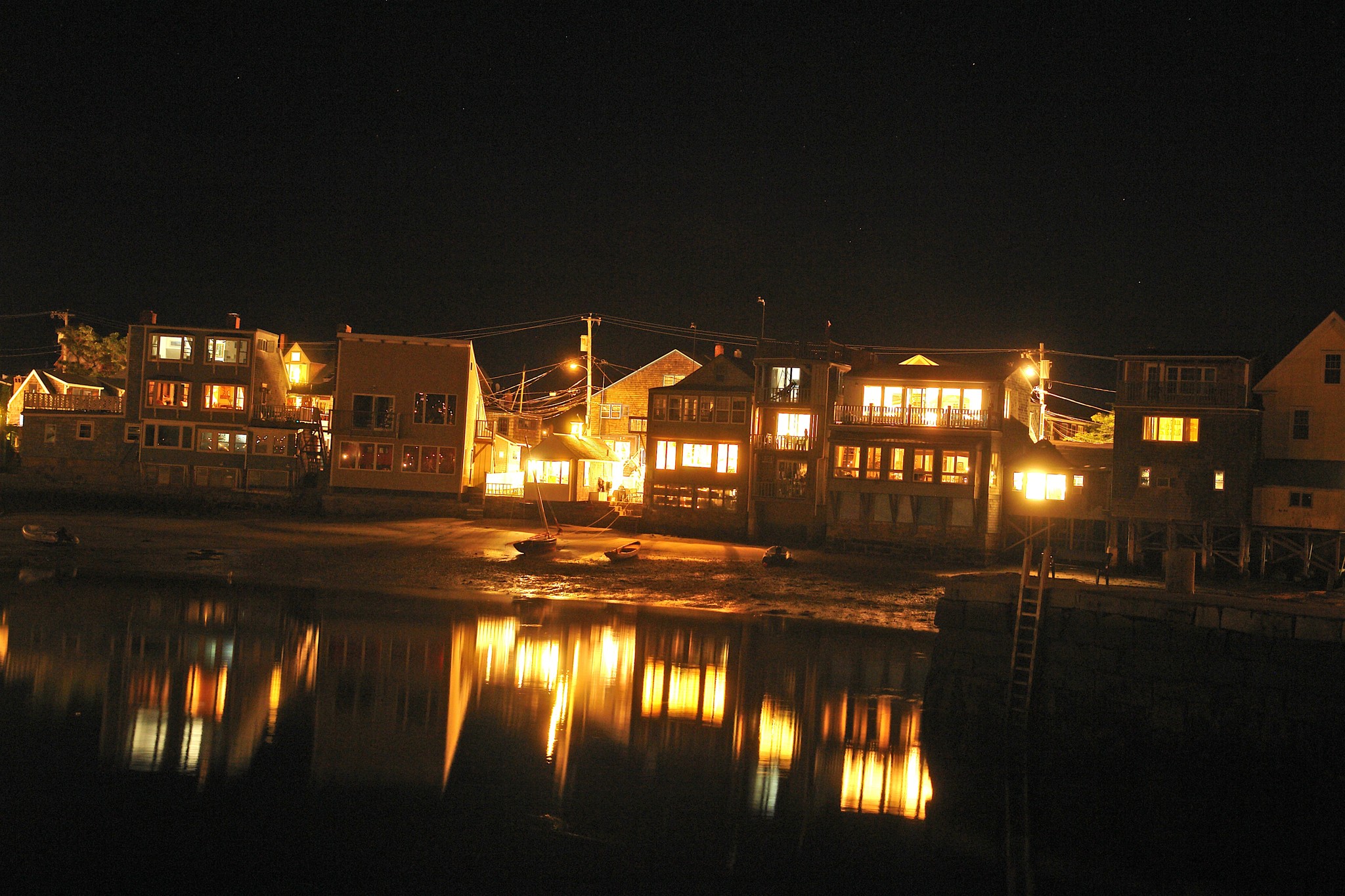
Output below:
[513,435,557,554]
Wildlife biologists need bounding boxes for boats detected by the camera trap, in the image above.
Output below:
[21,524,80,546]
[761,545,797,569]
[604,540,642,560]
